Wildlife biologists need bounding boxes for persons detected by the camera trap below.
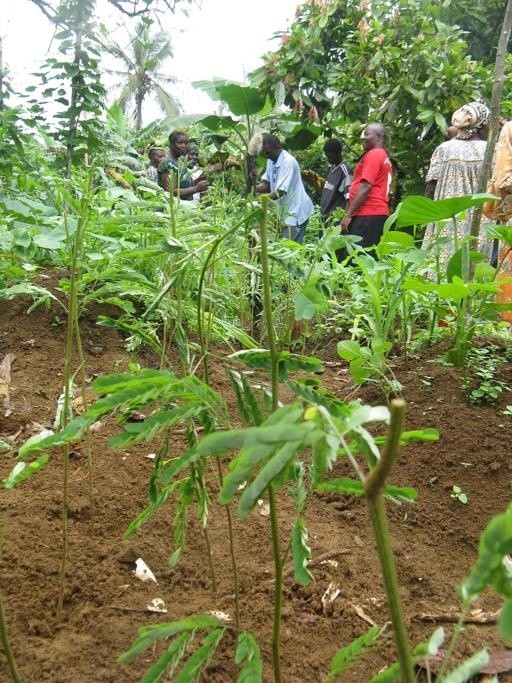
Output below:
[340,122,393,278]
[415,99,501,309]
[148,129,241,205]
[479,120,511,321]
[253,133,315,246]
[319,137,352,241]
